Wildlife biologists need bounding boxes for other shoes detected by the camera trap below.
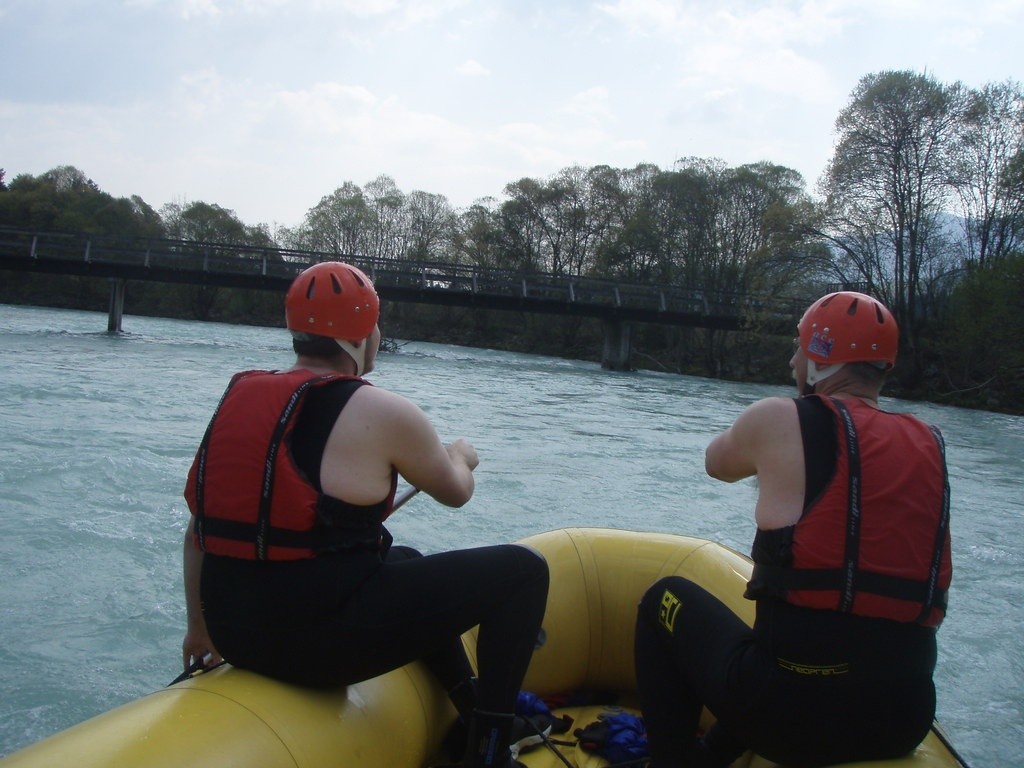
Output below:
[507,714,552,759]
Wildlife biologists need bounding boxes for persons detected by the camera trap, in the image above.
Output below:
[181,261,552,768]
[604,289,953,768]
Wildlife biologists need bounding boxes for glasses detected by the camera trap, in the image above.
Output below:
[791,338,806,360]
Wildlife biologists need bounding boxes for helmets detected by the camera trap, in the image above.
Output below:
[283,261,380,342]
[798,291,900,366]
